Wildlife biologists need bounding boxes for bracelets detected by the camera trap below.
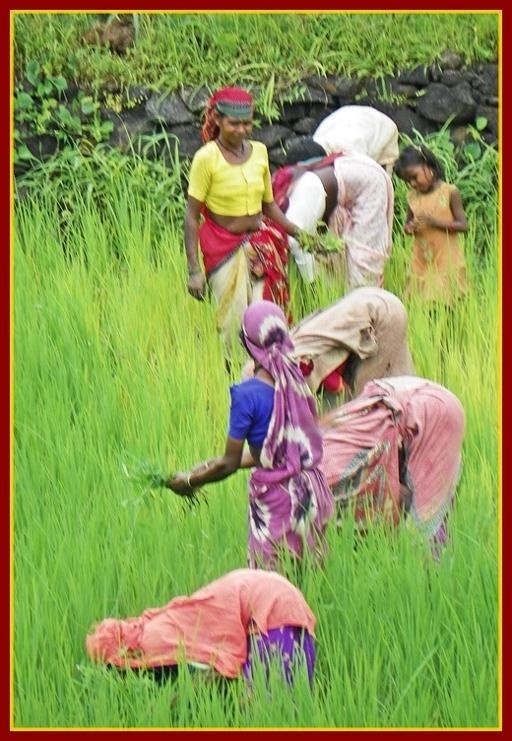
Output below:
[187,268,203,278]
[204,463,209,470]
[187,475,193,489]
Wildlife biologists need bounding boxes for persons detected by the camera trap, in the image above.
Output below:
[160,300,338,587]
[253,151,396,290]
[311,375,468,556]
[280,103,401,179]
[237,285,420,402]
[82,566,320,702]
[182,86,331,380]
[390,145,473,309]
